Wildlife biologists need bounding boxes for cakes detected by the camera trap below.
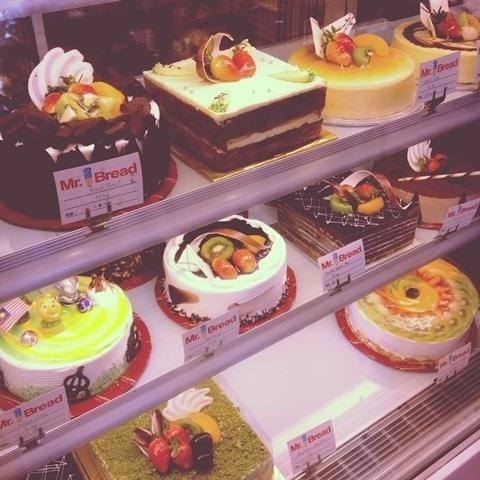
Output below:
[160,212,290,324]
[344,258,480,366]
[85,244,163,292]
[139,32,328,171]
[276,172,422,269]
[18,452,83,480]
[377,135,480,230]
[83,375,277,480]
[0,272,142,407]
[1,47,171,219]
[281,12,419,120]
[392,0,480,89]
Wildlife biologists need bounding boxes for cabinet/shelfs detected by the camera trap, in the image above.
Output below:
[0,1,480,479]
